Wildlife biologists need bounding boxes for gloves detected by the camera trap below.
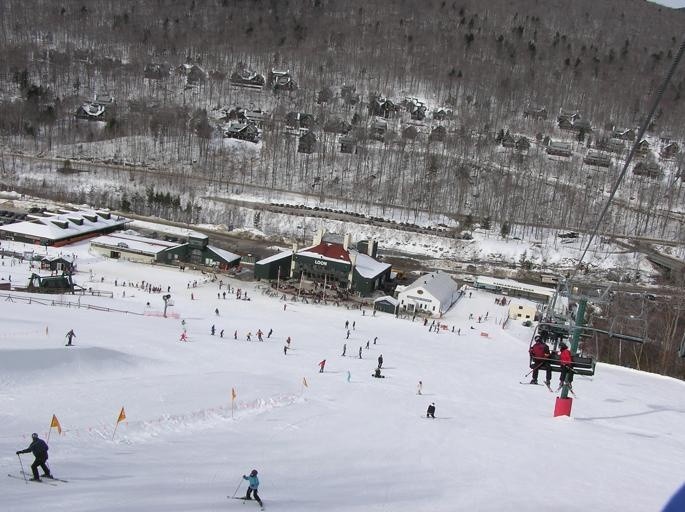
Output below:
[16,451,21,454]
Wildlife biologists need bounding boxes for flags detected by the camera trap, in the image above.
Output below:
[117,409,128,425]
[231,389,236,401]
[50,414,62,432]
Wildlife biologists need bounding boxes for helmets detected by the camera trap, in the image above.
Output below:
[559,343,566,350]
[252,470,257,475]
[32,432,37,438]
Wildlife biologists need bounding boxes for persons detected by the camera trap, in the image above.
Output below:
[64,328,75,346]
[14,432,49,481]
[282,342,386,386]
[0,238,94,296]
[426,401,436,418]
[180,333,187,341]
[558,342,574,389]
[100,263,510,342]
[416,380,423,396]
[528,334,552,388]
[242,468,264,511]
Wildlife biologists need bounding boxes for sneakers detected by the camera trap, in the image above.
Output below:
[531,379,537,383]
[567,381,572,388]
[546,380,550,384]
[560,381,564,386]
[30,477,38,480]
[42,474,50,476]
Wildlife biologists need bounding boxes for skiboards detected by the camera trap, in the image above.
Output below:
[7,470,69,488]
[520,381,555,392]
[226,495,266,511]
[555,384,579,399]
